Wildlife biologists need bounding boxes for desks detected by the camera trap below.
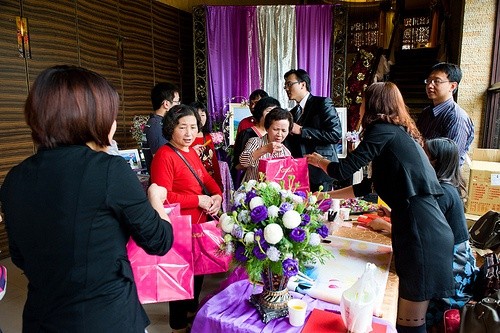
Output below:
[192,200,401,333]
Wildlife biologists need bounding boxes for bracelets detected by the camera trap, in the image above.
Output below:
[318,157,328,167]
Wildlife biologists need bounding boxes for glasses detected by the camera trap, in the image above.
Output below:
[283,81,299,89]
[424,78,453,85]
[173,100,181,104]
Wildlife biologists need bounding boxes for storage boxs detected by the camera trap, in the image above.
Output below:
[465,148,500,217]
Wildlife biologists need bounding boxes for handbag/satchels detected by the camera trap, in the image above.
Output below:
[477,253,500,299]
[259,145,310,196]
[191,208,232,275]
[122,203,194,304]
[468,210,500,249]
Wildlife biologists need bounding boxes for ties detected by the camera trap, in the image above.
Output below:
[296,106,303,125]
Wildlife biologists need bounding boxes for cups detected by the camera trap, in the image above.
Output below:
[340,208,350,221]
[332,199,341,211]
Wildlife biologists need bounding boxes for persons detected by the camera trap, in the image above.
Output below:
[0,66,174,333]
[145,62,476,333]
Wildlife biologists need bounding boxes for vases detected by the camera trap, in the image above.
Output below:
[248,286,293,324]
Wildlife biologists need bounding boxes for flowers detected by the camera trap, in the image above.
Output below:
[209,131,226,150]
[218,171,335,292]
